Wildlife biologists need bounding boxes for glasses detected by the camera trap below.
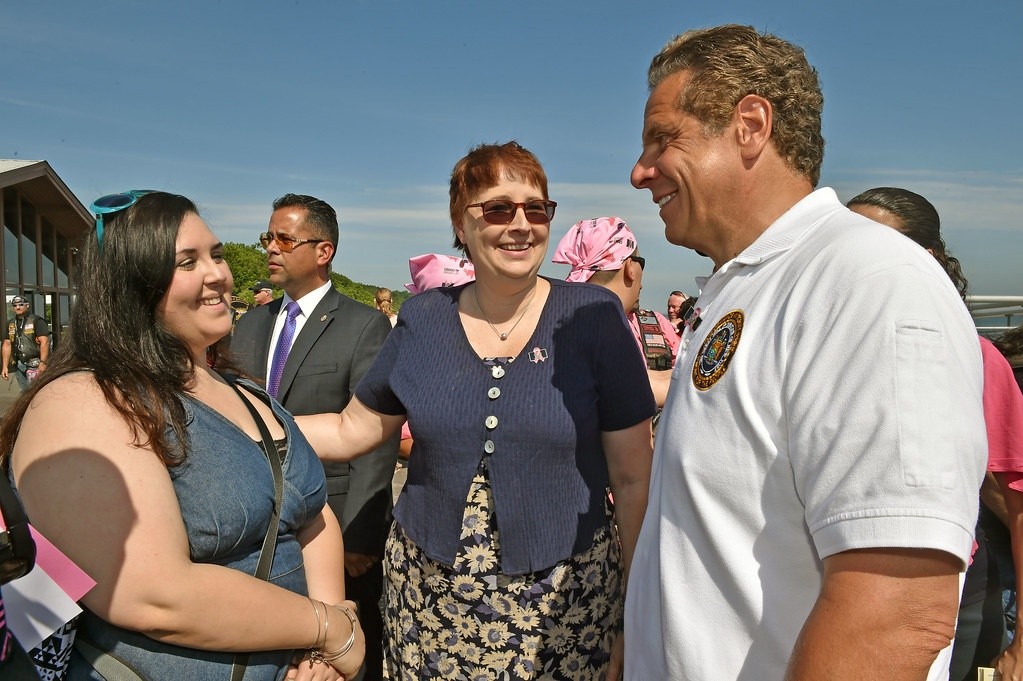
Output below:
[259,230,325,253]
[627,255,645,271]
[90,190,159,255]
[670,291,686,300]
[12,304,26,307]
[463,198,557,224]
[254,289,271,294]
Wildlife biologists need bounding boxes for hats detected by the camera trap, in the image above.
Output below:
[12,296,29,303]
[248,280,272,289]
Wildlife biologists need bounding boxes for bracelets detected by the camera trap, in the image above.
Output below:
[305,597,356,665]
[40,360,46,365]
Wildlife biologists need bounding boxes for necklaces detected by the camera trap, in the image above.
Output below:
[475,288,537,340]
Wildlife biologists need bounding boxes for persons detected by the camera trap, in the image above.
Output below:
[551,216,646,318]
[847,187,1022,681]
[667,291,690,337]
[248,281,278,307]
[374,287,397,328]
[0,189,366,681]
[398,252,476,460]
[625,24,988,681]
[625,283,680,448]
[1,295,50,390]
[225,194,402,681]
[293,136,658,680]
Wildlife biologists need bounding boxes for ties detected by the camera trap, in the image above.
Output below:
[267,301,302,400]
